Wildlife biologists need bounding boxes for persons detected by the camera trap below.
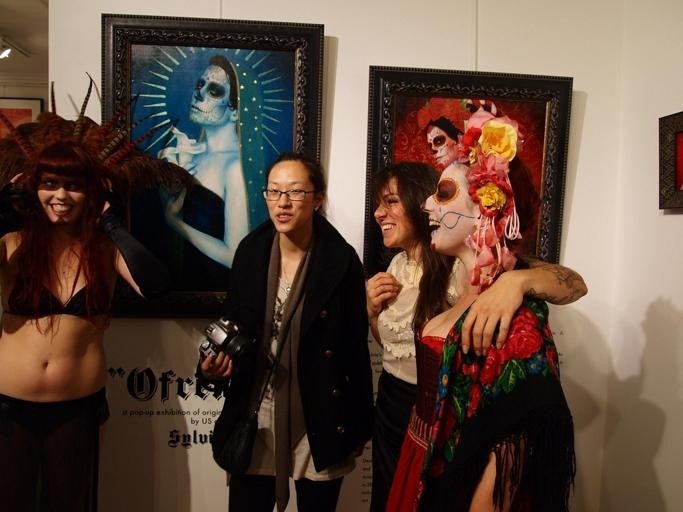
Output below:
[157,51,269,293]
[1,140,173,511]
[194,153,378,512]
[425,119,473,173]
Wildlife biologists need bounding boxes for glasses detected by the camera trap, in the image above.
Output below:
[262,187,316,205]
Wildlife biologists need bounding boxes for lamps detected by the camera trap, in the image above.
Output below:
[0,47,12,61]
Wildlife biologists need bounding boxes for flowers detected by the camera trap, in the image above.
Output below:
[460,115,521,217]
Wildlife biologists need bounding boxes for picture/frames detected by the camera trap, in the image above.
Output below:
[658,111,683,209]
[361,65,574,320]
[100,13,325,322]
[0,97,44,138]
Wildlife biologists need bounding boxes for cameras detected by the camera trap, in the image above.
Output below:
[199,317,253,363]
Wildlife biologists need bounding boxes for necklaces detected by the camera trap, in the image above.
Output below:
[280,264,297,295]
